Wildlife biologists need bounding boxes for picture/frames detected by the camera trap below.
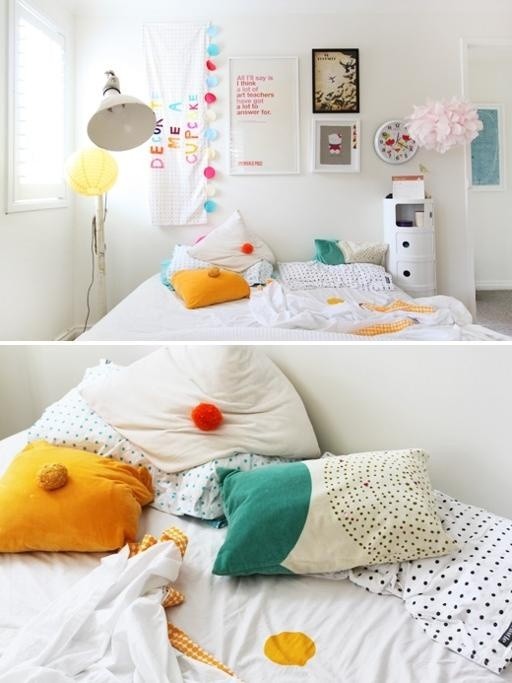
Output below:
[229,46,361,173]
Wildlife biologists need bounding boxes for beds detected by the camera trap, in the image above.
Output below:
[0,422,512,683]
[76,261,510,342]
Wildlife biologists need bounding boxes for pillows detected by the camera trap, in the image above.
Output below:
[77,345,323,476]
[308,447,510,673]
[212,441,460,578]
[28,386,299,529]
[0,437,154,556]
[161,210,388,309]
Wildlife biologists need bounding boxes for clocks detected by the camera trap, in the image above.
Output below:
[375,120,417,162]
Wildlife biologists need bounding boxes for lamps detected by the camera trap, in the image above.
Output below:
[58,70,156,341]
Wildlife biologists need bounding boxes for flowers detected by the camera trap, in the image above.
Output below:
[408,94,483,151]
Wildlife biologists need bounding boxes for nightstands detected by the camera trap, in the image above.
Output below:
[384,194,438,297]
